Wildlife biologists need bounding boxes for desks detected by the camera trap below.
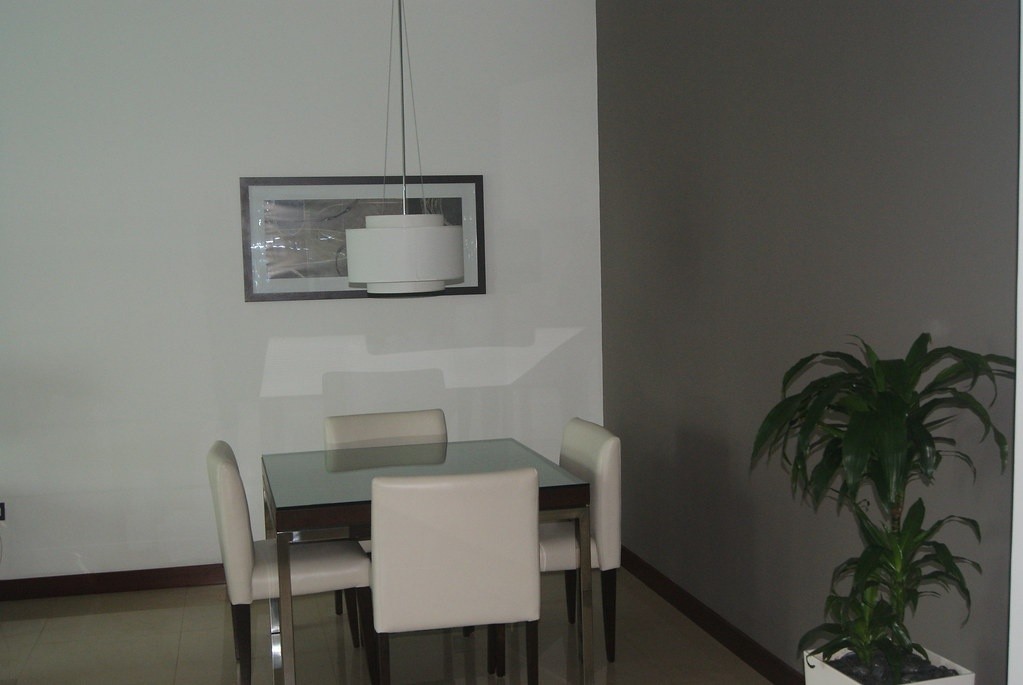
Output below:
[260,438,594,685]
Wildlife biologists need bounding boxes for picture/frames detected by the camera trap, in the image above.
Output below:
[239,176,487,302]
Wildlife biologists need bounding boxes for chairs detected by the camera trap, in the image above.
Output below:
[207,441,379,685]
[358,468,540,685]
[324,408,447,647]
[537,417,621,663]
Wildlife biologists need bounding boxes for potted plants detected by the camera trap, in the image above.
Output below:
[750,333,1016,685]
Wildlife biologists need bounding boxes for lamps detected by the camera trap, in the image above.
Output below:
[346,0,466,295]
